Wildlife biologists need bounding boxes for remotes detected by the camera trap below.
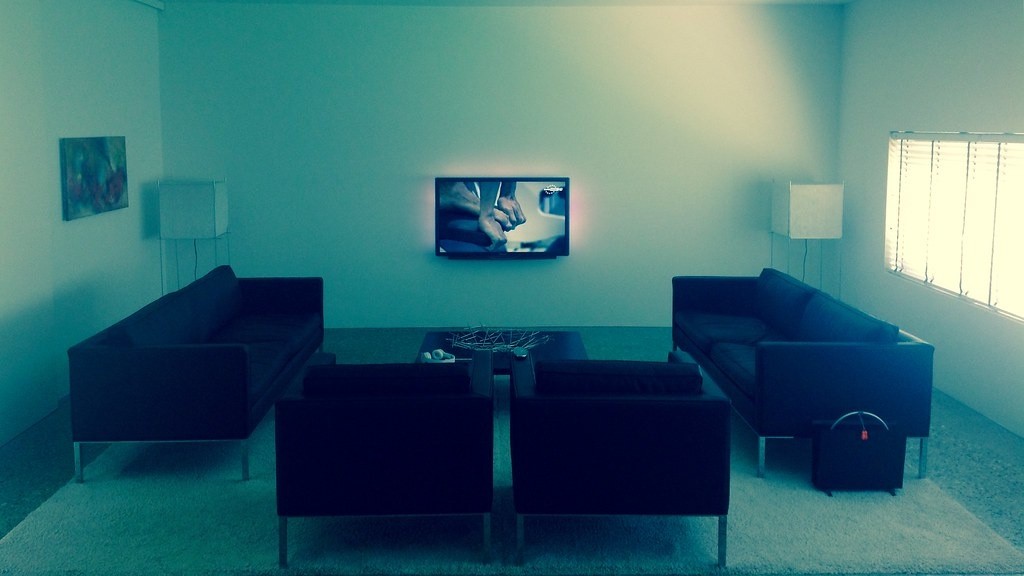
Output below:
[514,346,528,357]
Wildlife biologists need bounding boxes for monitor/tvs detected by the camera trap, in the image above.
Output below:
[434,177,569,259]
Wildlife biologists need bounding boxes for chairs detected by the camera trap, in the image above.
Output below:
[274,351,497,569]
[509,348,730,566]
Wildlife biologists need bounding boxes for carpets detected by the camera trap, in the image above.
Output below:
[1,327,1024,576]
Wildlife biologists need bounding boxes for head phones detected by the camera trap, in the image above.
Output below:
[422,348,455,364]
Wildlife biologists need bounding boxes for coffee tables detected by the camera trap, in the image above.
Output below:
[412,330,589,408]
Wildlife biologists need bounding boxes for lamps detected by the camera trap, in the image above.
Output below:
[771,177,844,304]
[158,176,230,298]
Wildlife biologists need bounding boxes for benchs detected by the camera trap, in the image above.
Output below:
[67,265,325,483]
[669,269,934,480]
